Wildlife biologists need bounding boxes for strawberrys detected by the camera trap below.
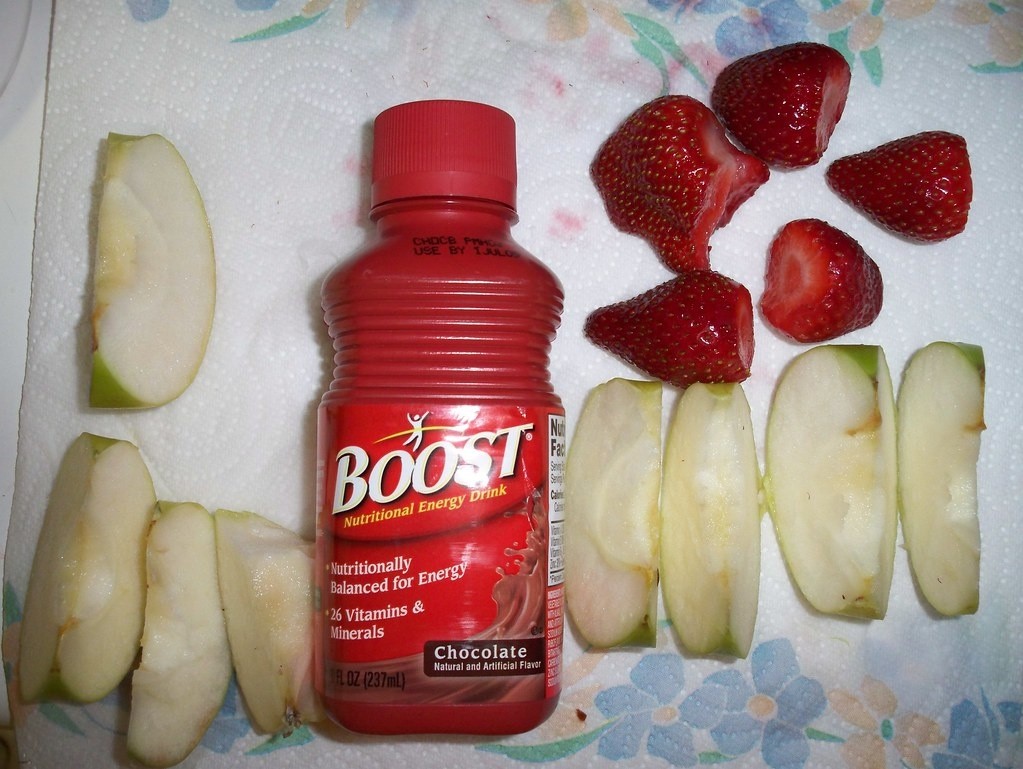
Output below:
[582,41,974,389]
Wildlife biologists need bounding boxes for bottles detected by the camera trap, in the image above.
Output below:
[313,96,564,734]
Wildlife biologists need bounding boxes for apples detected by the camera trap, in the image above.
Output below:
[18,132,322,768]
[556,343,982,656]
